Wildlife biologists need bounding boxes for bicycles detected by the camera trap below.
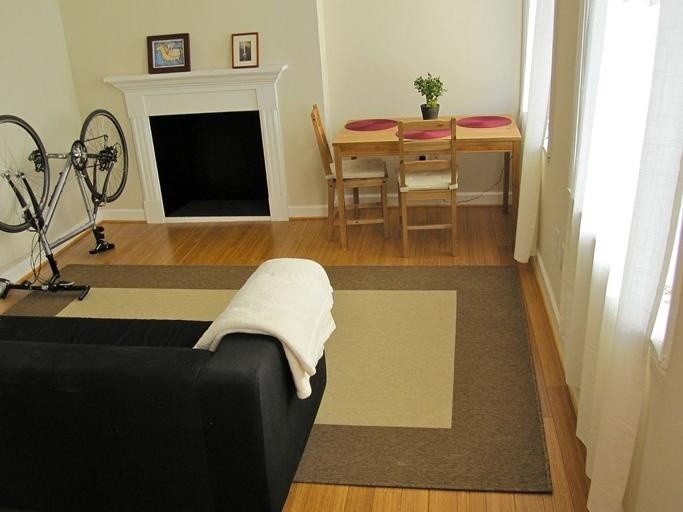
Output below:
[0,106,131,301]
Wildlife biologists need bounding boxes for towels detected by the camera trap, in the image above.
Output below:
[191,257,339,401]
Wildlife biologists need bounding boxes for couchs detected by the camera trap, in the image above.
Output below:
[0,259,327,511]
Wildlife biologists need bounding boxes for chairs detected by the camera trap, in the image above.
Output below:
[309,103,390,240]
[394,118,459,260]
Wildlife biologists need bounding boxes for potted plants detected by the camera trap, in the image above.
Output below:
[413,75,448,120]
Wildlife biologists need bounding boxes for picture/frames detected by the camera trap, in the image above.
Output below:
[146,32,191,74]
[231,31,260,69]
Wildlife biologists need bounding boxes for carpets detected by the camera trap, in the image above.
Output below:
[0,264,554,496]
